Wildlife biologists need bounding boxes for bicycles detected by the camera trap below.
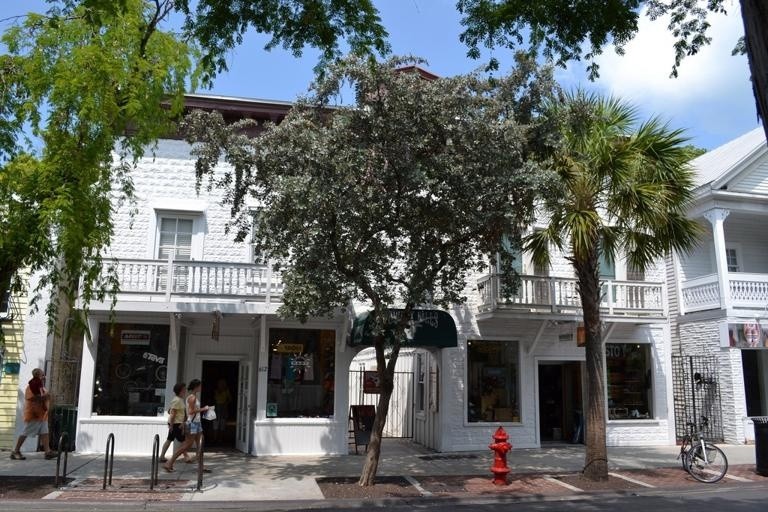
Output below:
[677,416,727,483]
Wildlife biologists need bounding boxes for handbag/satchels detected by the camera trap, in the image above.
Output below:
[181,423,191,436]
[202,405,217,421]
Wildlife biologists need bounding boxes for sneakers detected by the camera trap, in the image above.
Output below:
[45,451,58,460]
[10,451,26,460]
[158,456,213,474]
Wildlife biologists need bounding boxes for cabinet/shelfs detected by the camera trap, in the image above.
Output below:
[607,357,647,413]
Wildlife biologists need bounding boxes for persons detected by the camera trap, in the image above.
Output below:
[10,372,61,459]
[29,368,47,410]
[158,382,191,462]
[162,379,213,473]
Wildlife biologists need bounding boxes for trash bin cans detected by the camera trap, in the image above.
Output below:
[751,417,768,477]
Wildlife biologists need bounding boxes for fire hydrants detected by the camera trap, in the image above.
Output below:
[488,426,513,485]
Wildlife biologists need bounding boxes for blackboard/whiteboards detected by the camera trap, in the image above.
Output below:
[352,405,375,445]
